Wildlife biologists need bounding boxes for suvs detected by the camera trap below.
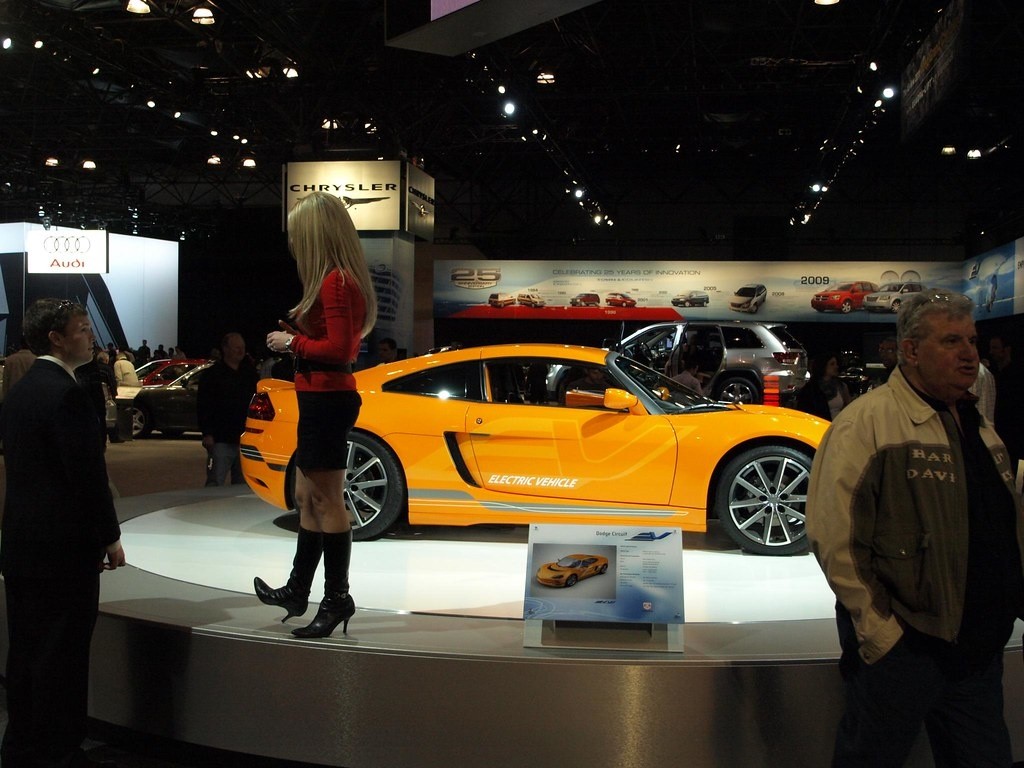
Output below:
[488,292,516,308]
[671,290,711,307]
[545,318,833,402]
[570,293,601,307]
[517,293,546,309]
[605,292,637,307]
[727,284,771,314]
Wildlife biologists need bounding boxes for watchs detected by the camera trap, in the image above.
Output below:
[285,336,297,351]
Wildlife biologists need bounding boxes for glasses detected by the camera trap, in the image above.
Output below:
[51,299,75,331]
[907,294,973,325]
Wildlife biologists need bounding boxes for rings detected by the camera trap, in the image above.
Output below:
[274,345,276,348]
[270,343,273,346]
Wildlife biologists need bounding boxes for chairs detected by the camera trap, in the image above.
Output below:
[686,330,699,360]
[523,363,549,405]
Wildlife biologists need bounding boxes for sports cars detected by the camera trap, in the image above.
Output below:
[533,553,611,590]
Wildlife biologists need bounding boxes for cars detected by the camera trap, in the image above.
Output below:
[861,282,929,315]
[231,344,836,559]
[132,358,213,389]
[131,361,218,439]
[810,281,880,314]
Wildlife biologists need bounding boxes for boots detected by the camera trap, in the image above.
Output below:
[254,525,323,623]
[290,525,355,637]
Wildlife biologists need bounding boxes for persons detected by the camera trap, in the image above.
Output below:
[112,352,140,386]
[2,334,37,397]
[138,340,150,363]
[255,192,378,636]
[796,353,852,422]
[805,286,1024,768]
[196,332,261,488]
[568,364,615,390]
[964,331,1024,487]
[878,336,899,367]
[669,358,712,396]
[378,338,398,363]
[95,352,126,444]
[0,298,128,767]
[152,344,188,375]
[107,342,115,357]
